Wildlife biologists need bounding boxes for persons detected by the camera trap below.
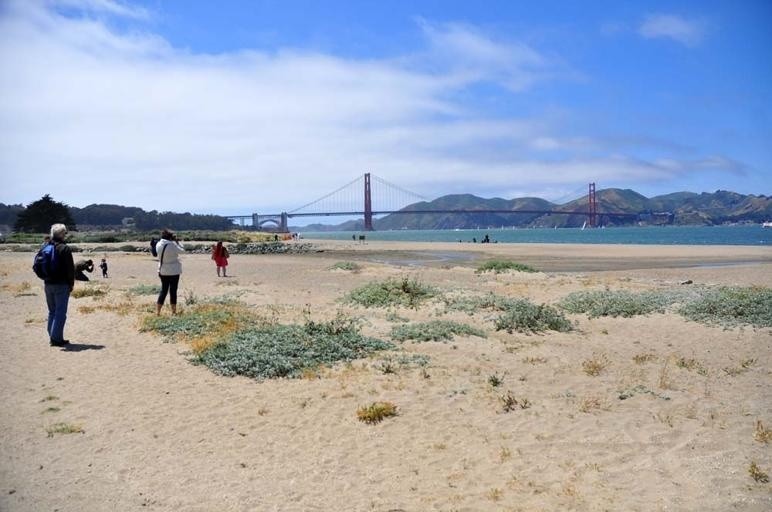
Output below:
[41,235,49,247]
[210,241,228,277]
[274,234,279,242]
[98,259,108,279]
[150,227,186,316]
[44,222,75,346]
[74,259,94,282]
[288,230,303,241]
[352,233,356,241]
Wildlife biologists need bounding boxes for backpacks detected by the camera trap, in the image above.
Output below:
[32,241,59,281]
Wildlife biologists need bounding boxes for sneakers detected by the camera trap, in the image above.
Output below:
[51,339,68,346]
